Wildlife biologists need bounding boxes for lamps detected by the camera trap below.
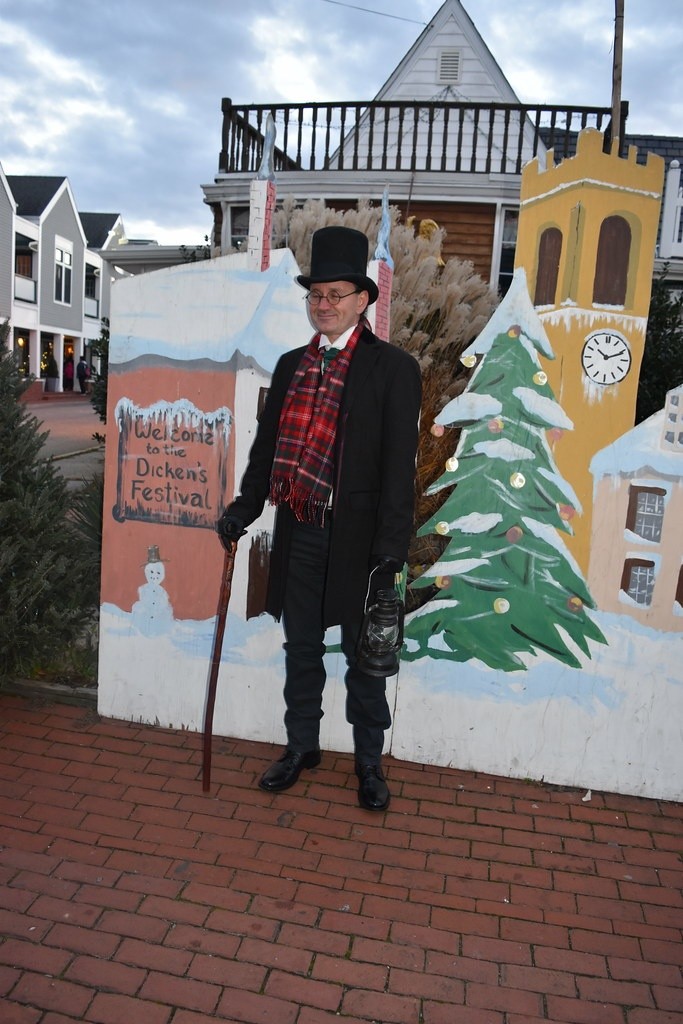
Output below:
[354,565,405,678]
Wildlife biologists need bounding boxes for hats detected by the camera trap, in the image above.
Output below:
[296,226,379,305]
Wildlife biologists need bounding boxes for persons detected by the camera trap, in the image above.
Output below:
[76,356,88,395]
[64,352,73,392]
[217,226,423,812]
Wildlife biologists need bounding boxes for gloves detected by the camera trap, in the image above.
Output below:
[217,512,248,552]
[369,555,404,576]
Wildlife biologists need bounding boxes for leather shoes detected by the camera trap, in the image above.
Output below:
[354,761,390,811]
[258,744,321,791]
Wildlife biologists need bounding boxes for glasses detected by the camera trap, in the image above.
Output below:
[306,289,358,305]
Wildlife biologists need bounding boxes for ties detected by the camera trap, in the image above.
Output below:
[323,348,340,373]
